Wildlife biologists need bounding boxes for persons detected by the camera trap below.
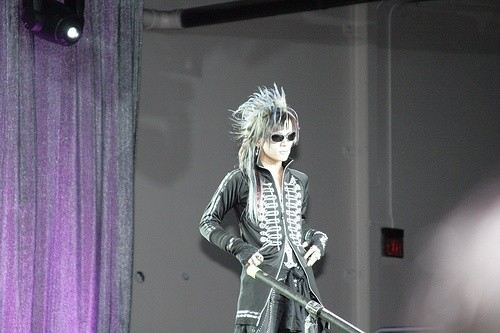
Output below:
[200,82,330,333]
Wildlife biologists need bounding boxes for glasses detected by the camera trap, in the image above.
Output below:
[265,128,297,144]
